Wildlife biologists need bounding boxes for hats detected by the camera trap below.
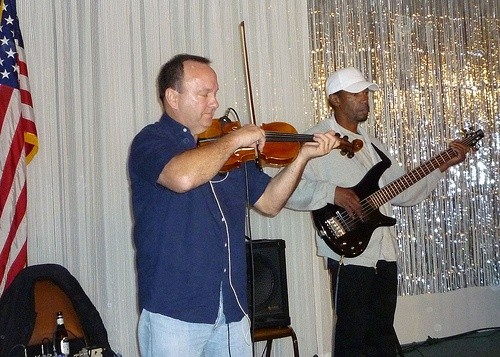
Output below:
[325,67,379,99]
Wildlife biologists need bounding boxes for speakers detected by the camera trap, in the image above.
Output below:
[245,238,291,330]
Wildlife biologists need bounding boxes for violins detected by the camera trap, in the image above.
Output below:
[196,119,363,173]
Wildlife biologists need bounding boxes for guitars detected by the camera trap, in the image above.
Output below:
[311,126,485,258]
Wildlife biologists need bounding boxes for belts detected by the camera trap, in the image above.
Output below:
[377,260,396,270]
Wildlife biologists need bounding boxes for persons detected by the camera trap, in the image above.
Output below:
[284,66,470,357]
[124,52,341,357]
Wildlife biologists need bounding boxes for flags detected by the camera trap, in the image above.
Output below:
[0,0,39,299]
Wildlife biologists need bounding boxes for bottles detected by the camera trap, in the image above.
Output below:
[55,311,70,357]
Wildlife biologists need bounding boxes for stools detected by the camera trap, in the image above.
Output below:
[252,327,299,357]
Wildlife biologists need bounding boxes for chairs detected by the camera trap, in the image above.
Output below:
[0,263,118,357]
